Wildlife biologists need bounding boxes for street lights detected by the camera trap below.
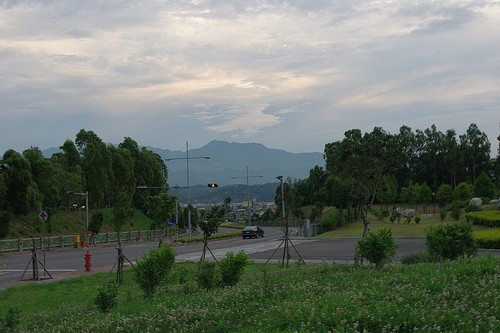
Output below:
[275,174,285,220]
[164,142,212,239]
[66,191,89,247]
[137,184,179,242]
[231,166,264,226]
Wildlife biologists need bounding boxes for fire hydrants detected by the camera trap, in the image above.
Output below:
[83,250,92,272]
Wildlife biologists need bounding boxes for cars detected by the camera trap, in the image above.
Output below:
[241,226,264,239]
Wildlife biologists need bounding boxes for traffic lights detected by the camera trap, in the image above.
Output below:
[208,183,219,188]
